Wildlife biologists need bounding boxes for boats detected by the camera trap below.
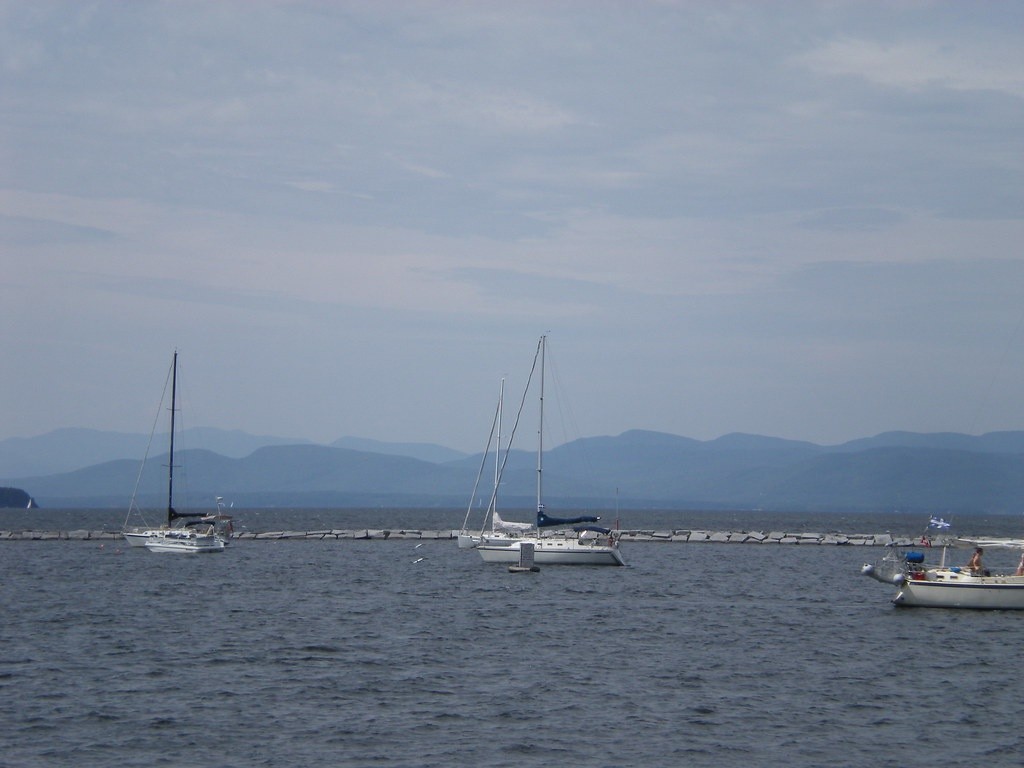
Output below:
[143,521,227,554]
[858,549,1024,611]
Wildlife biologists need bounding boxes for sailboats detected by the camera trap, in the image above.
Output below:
[120,350,212,548]
[452,334,628,566]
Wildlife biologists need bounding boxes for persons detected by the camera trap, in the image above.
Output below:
[966,547,991,578]
